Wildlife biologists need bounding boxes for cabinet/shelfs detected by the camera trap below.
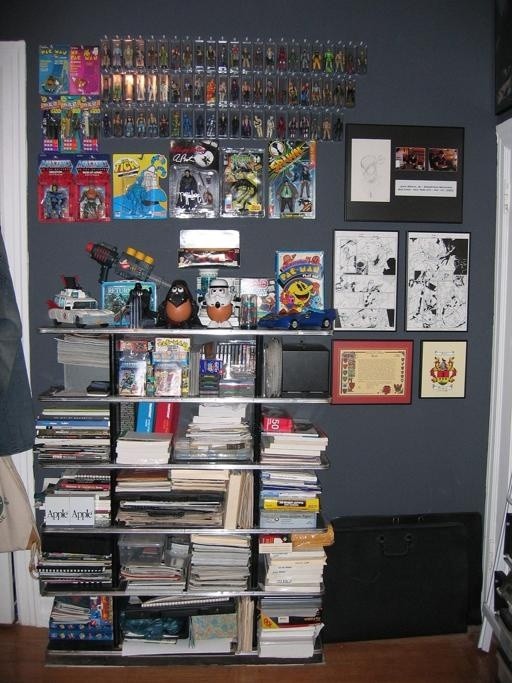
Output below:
[34,324,336,669]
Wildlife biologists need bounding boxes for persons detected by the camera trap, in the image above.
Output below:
[39,35,365,215]
[125,282,159,324]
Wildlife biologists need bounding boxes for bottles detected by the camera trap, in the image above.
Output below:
[240,293,258,327]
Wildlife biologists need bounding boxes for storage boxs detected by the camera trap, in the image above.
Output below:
[113,532,170,565]
[282,340,329,397]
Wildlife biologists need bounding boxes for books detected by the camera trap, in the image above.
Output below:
[32,340,333,659]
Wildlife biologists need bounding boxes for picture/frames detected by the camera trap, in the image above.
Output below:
[341,121,468,222]
[328,336,416,407]
[331,226,398,335]
[416,337,470,401]
[401,229,475,333]
